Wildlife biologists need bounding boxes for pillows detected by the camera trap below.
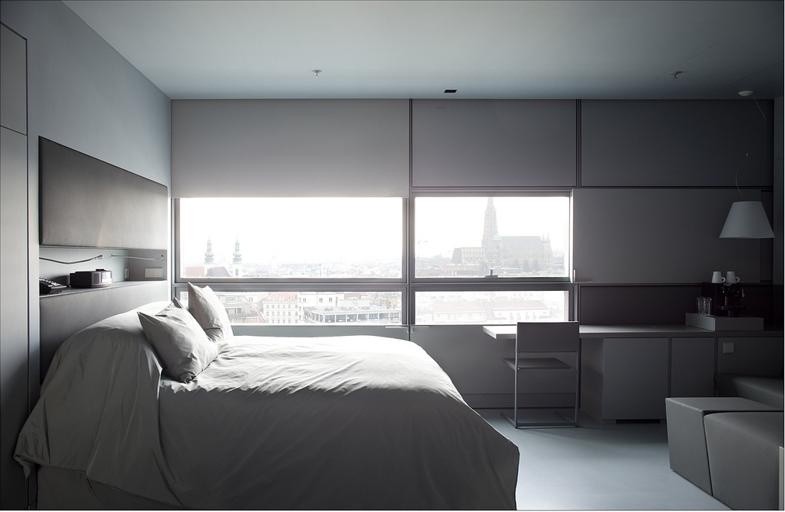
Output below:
[188,281,234,355]
[137,296,219,383]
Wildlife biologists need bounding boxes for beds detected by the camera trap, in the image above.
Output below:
[13,299,520,510]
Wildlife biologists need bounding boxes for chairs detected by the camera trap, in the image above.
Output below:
[501,322,579,428]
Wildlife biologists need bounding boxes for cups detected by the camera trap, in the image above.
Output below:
[725,270,741,284]
[697,297,712,316]
[711,271,725,284]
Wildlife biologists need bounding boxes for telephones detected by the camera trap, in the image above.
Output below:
[39,278,67,295]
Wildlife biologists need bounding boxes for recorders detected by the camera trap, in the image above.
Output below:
[70,269,113,288]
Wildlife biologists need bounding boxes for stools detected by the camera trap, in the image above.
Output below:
[665,396,784,510]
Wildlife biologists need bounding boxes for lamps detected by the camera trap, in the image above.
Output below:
[718,91,776,239]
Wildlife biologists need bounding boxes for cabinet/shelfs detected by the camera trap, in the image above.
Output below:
[581,337,716,424]
[570,188,772,284]
[717,337,784,397]
